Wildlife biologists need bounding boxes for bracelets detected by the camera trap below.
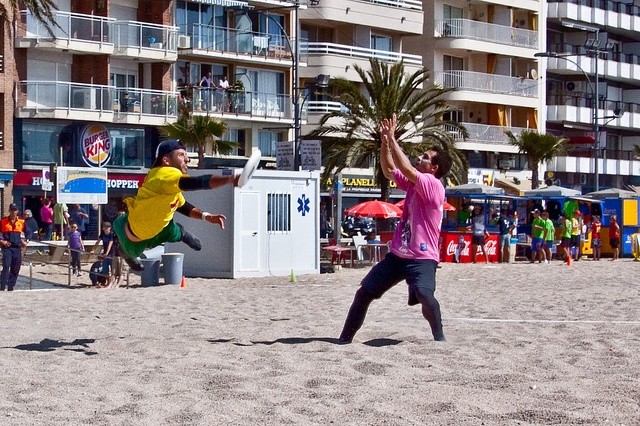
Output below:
[202,211,211,223]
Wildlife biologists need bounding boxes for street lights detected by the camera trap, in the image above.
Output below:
[225,0,330,170]
[534,30,624,190]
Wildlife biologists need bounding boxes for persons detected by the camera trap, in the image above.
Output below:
[69,204,89,240]
[1,203,32,292]
[463,205,622,265]
[35,198,54,255]
[339,113,452,341]
[66,221,86,277]
[199,72,218,110]
[113,139,241,271]
[22,210,40,255]
[53,203,70,240]
[90,221,115,288]
[216,76,232,112]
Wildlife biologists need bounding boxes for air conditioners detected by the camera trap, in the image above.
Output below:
[565,80,583,92]
[177,35,191,49]
[71,89,96,109]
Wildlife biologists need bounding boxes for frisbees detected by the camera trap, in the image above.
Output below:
[237,150,261,188]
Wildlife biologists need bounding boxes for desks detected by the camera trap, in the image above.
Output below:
[357,243,393,266]
[515,239,561,261]
[321,244,352,269]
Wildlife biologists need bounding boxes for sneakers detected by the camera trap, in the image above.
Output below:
[37,250,42,255]
[78,273,82,276]
[125,258,144,271]
[74,272,77,275]
[41,251,49,256]
[176,223,201,251]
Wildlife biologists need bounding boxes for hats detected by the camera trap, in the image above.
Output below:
[103,222,111,227]
[23,210,32,215]
[610,215,616,220]
[592,215,600,223]
[150,139,186,170]
[69,224,78,230]
[10,203,18,210]
[575,210,582,216]
[531,209,541,214]
